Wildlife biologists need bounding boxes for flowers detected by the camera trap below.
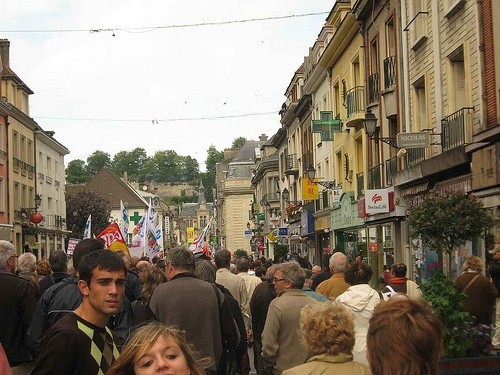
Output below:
[420,268,496,358]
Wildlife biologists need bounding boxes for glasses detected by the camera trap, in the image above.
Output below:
[274,277,284,282]
[6,255,18,260]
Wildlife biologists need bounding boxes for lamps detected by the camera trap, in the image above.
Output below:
[281,188,298,205]
[363,110,400,150]
[246,221,257,233]
[21,194,42,213]
[307,164,335,190]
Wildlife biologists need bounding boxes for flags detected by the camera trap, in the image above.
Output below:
[189,222,224,259]
[82,203,162,262]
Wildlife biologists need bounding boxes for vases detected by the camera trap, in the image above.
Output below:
[438,358,500,375]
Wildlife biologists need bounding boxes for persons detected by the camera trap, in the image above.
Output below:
[488,245,500,349]
[453,255,497,356]
[0,238,252,375]
[250,252,445,375]
